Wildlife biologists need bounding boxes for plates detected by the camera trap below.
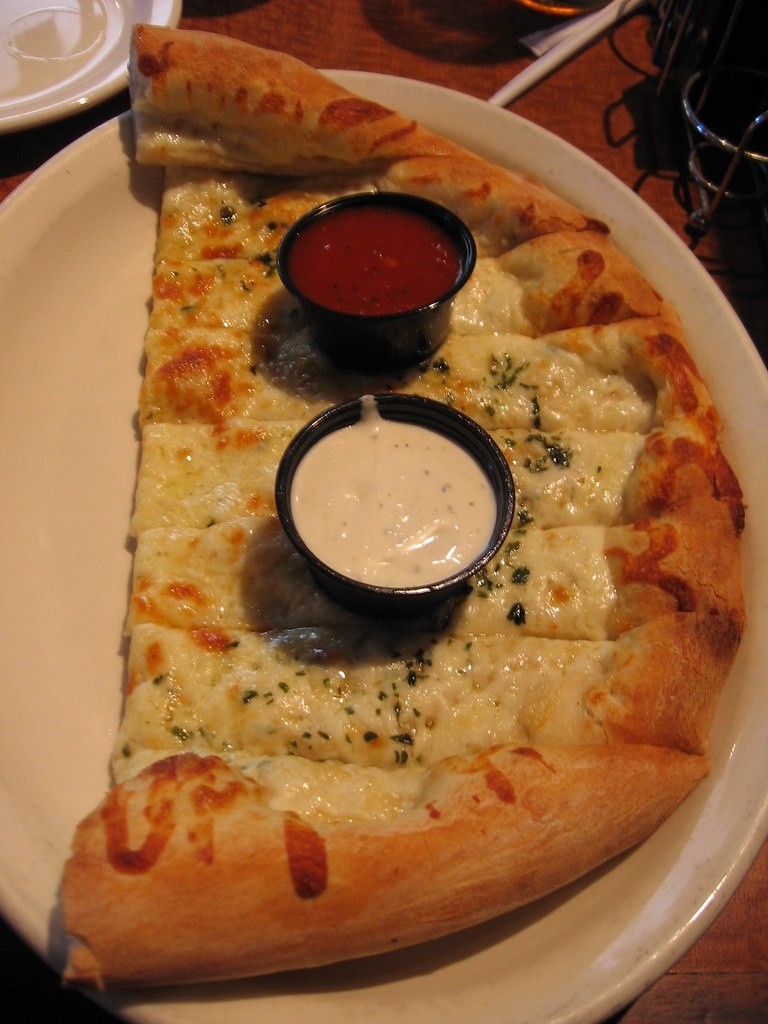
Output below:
[0,63,768,1023]
[0,1,185,136]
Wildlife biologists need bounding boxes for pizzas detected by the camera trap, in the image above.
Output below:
[63,24,745,982]
